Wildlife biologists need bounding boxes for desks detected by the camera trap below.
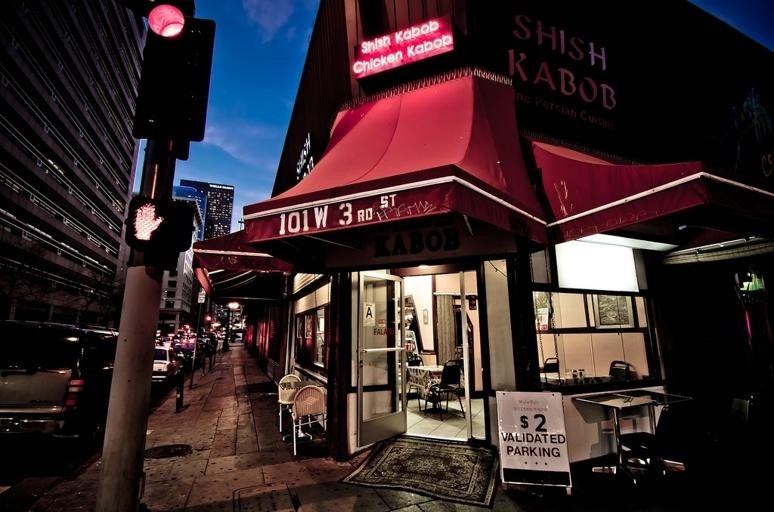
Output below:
[572,390,692,475]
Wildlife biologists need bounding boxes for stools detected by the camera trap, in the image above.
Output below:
[619,432,664,486]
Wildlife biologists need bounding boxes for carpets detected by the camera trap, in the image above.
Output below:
[338,435,500,508]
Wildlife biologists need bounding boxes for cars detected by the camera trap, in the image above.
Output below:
[0,318,114,453]
[152,329,218,385]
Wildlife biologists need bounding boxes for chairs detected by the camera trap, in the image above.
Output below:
[407,356,465,421]
[609,360,630,379]
[539,358,560,380]
[277,374,326,456]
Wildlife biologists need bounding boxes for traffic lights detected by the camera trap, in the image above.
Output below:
[134,1,215,145]
[124,192,196,273]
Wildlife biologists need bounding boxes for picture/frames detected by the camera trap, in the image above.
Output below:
[591,294,635,330]
